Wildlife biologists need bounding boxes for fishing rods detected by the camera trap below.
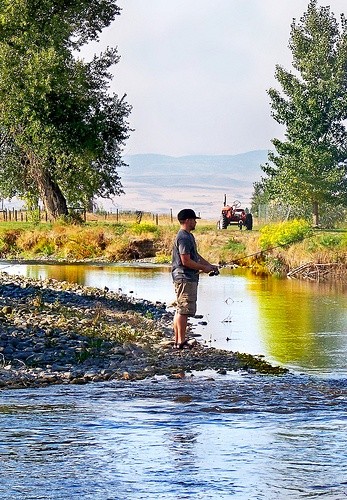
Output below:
[196,229,325,276]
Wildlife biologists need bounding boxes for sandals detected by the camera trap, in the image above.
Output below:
[173,343,177,349]
[176,341,197,350]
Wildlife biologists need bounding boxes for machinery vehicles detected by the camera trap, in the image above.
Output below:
[216,199,252,230]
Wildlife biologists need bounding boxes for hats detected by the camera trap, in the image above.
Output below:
[177,209,202,220]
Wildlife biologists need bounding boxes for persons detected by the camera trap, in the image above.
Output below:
[169,208,218,350]
